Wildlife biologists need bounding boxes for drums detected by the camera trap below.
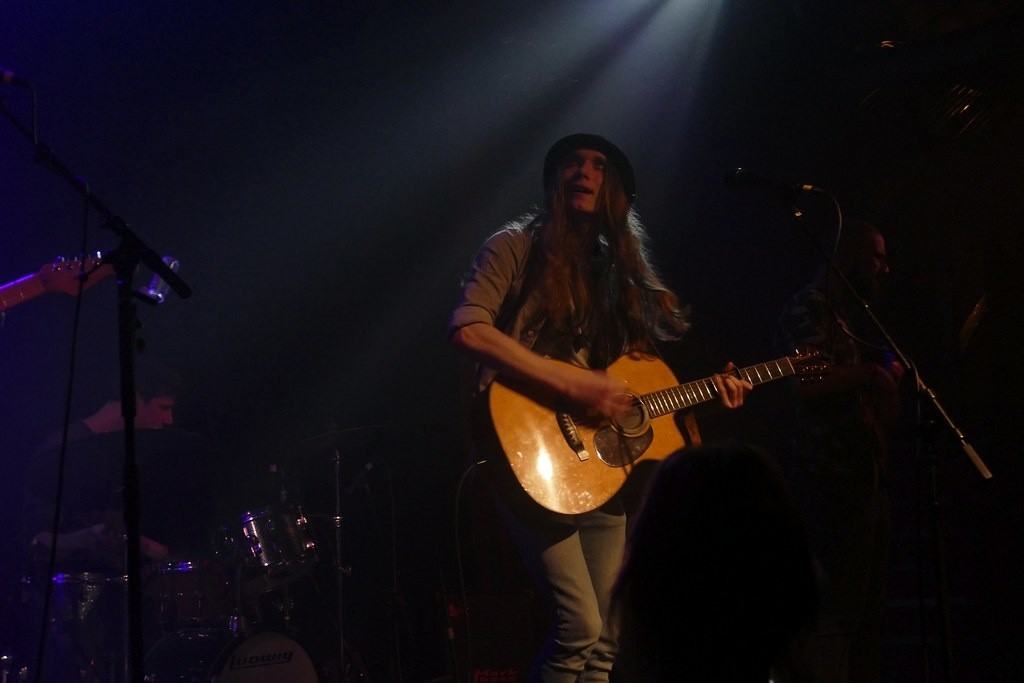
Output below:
[53,570,126,652]
[212,501,320,600]
[159,559,220,630]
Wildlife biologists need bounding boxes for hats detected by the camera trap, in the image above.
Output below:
[543,132,636,207]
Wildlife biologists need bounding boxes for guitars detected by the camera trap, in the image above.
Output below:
[475,343,835,531]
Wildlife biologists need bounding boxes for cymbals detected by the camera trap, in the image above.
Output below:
[294,424,392,452]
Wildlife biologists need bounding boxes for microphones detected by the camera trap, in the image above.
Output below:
[724,167,814,193]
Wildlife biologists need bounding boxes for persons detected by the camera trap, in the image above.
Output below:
[447,134,758,683]
[25,352,192,565]
[776,221,929,680]
